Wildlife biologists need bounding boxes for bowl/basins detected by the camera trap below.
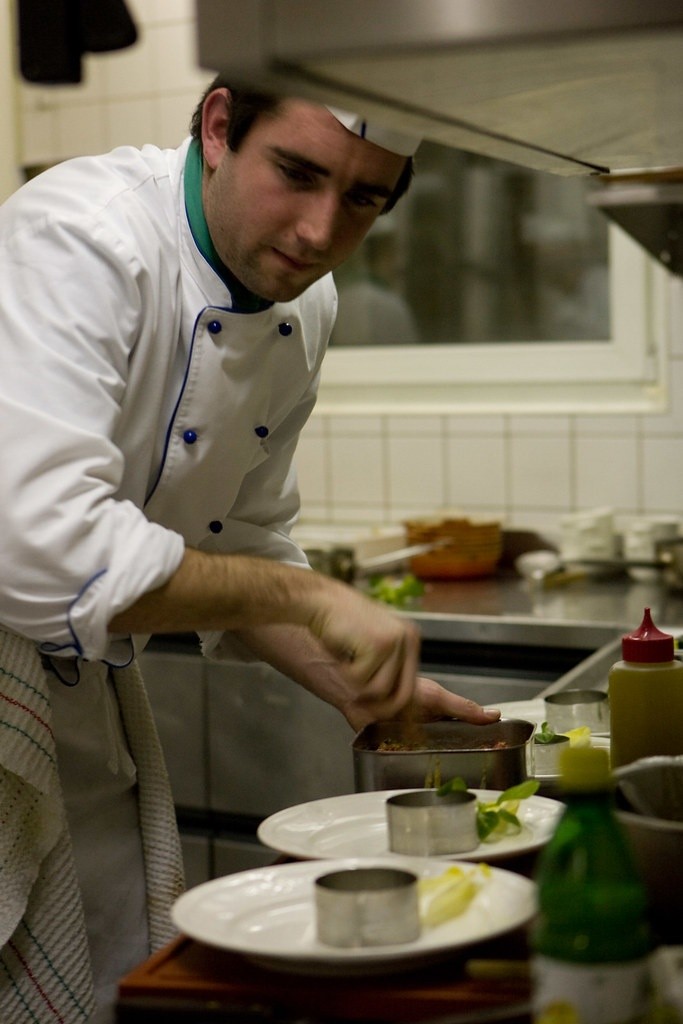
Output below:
[603,753,683,901]
[403,519,503,580]
[556,511,680,584]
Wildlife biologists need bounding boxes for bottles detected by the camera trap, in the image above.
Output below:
[608,607,683,771]
[530,748,655,1024]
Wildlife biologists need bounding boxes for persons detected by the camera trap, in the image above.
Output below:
[0,68,503,1024]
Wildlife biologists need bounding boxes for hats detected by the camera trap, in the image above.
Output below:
[327,104,422,155]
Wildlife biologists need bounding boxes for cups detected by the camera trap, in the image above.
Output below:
[544,690,609,734]
[313,866,420,948]
[384,790,476,854]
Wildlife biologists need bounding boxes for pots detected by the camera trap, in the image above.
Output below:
[560,539,683,594]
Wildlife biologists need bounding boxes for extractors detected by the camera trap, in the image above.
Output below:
[196,1,682,280]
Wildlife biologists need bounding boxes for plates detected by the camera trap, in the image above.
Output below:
[481,700,547,734]
[525,736,611,795]
[170,857,540,978]
[256,788,568,864]
